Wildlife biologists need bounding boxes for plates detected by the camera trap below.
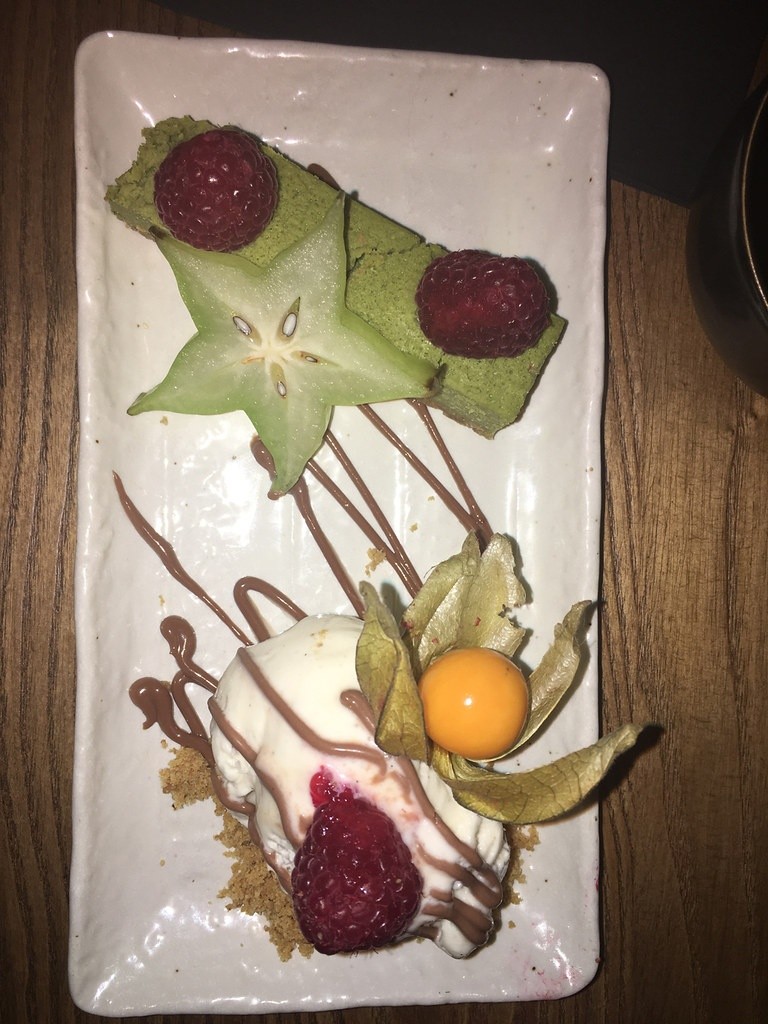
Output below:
[65,30,609,1020]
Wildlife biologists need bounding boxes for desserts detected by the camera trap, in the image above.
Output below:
[104,112,646,956]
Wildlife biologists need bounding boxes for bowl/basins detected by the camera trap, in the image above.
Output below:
[692,88,768,320]
